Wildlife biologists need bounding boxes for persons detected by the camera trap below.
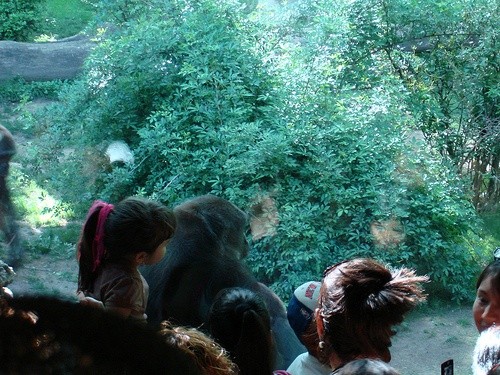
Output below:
[285,280,336,375]
[314,258,431,375]
[76,198,178,322]
[206,287,292,375]
[473,260,500,336]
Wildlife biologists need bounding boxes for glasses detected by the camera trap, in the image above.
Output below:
[323,260,349,278]
[494,248,500,260]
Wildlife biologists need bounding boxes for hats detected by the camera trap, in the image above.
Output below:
[287,281,323,340]
